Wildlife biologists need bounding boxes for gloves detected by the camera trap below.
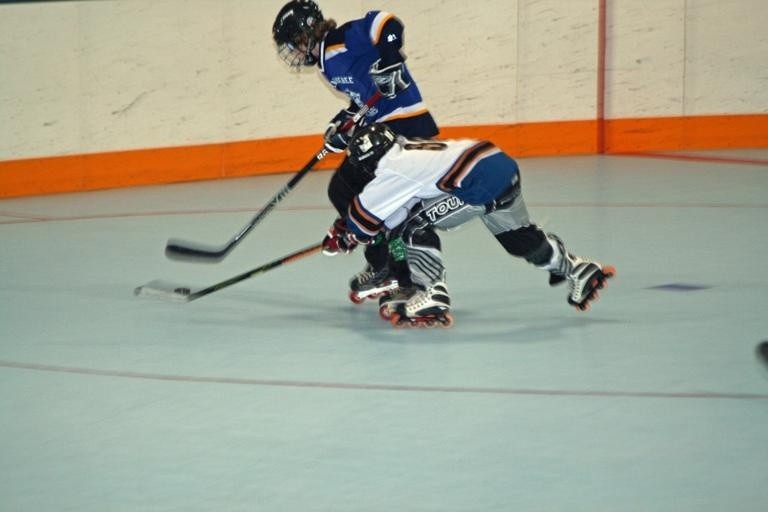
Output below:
[323,108,359,153]
[322,218,370,256]
[369,53,410,101]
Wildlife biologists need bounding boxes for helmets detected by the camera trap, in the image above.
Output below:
[272,0,324,74]
[346,124,397,182]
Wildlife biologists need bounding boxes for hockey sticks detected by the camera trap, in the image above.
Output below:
[134,243,323,302]
[165,92,382,262]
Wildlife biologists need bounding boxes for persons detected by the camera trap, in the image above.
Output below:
[272,0,440,292]
[323,123,601,319]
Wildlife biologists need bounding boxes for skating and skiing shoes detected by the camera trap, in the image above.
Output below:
[379,282,453,330]
[350,264,400,304]
[567,253,616,312]
